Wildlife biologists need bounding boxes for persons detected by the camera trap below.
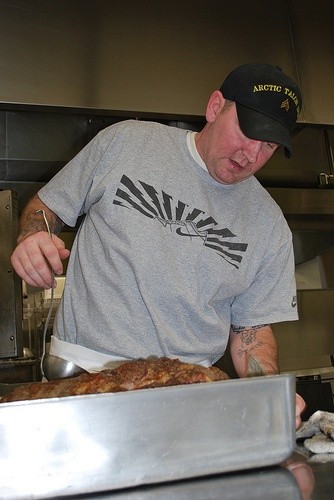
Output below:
[10,62,306,431]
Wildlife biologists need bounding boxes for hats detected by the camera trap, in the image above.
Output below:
[221,63,303,158]
